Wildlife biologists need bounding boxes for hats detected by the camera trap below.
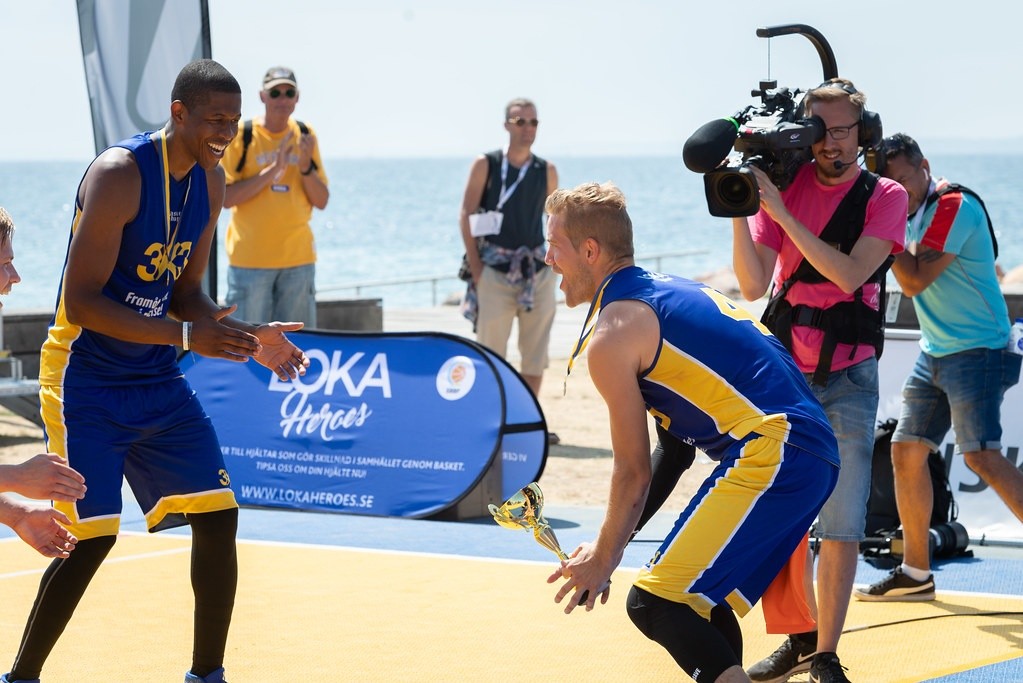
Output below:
[261,68,297,89]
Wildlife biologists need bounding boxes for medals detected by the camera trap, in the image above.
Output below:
[564,381,566,396]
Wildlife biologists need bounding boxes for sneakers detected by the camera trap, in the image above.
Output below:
[856,566,936,602]
[746,634,817,681]
[810,652,848,682]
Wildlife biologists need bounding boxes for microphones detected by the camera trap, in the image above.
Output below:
[682,118,740,174]
[834,147,868,169]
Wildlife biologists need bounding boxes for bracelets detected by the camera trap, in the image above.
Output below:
[300,166,312,176]
[183,322,193,350]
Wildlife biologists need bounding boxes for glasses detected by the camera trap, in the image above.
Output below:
[506,116,539,128]
[264,89,296,99]
[827,121,860,141]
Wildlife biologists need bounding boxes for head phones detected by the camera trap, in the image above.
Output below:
[823,84,883,151]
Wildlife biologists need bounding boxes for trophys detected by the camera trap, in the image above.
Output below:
[488,482,612,607]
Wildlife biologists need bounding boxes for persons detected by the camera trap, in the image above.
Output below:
[732,77,909,683]
[461,98,559,445]
[545,180,842,683]
[221,67,330,329]
[855,133,1023,603]
[0,59,310,683]
[0,208,86,558]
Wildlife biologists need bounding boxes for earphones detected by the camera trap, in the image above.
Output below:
[923,168,929,181]
